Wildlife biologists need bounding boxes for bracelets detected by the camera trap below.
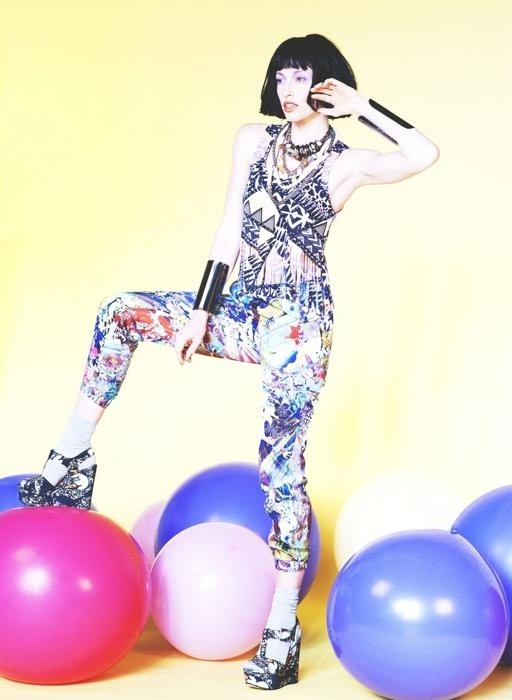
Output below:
[358,98,415,146]
[192,260,229,315]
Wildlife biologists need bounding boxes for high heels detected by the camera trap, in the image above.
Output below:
[18,448,96,510]
[243,616,301,690]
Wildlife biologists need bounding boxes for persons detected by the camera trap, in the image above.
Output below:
[20,34,440,690]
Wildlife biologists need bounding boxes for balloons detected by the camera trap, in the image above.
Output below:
[0,473,97,516]
[0,507,151,685]
[326,530,512,700]
[453,485,512,667]
[147,522,277,661]
[154,462,320,605]
